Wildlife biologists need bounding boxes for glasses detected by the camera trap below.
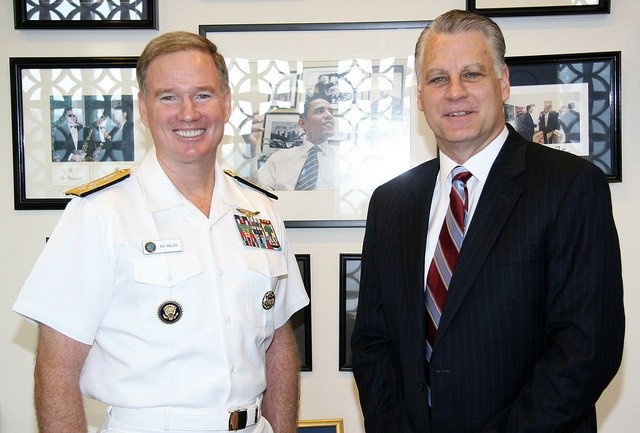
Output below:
[67,114,77,117]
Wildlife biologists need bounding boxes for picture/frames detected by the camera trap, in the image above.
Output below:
[13,0,157,29]
[506,50,622,184]
[199,20,433,228]
[464,0,611,19]
[297,419,343,433]
[9,57,142,209]
[286,253,312,370]
[339,253,363,371]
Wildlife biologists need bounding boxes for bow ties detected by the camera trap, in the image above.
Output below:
[70,125,78,128]
[100,125,106,130]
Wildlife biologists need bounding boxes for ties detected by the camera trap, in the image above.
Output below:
[295,147,321,190]
[424,164,474,421]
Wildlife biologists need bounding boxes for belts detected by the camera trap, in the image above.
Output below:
[109,394,274,430]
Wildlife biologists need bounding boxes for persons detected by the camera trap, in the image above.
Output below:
[559,102,580,143]
[312,74,339,102]
[90,111,113,161]
[54,107,92,161]
[258,98,355,189]
[351,10,625,433]
[103,105,132,160]
[523,105,538,141]
[539,103,557,143]
[11,31,310,432]
